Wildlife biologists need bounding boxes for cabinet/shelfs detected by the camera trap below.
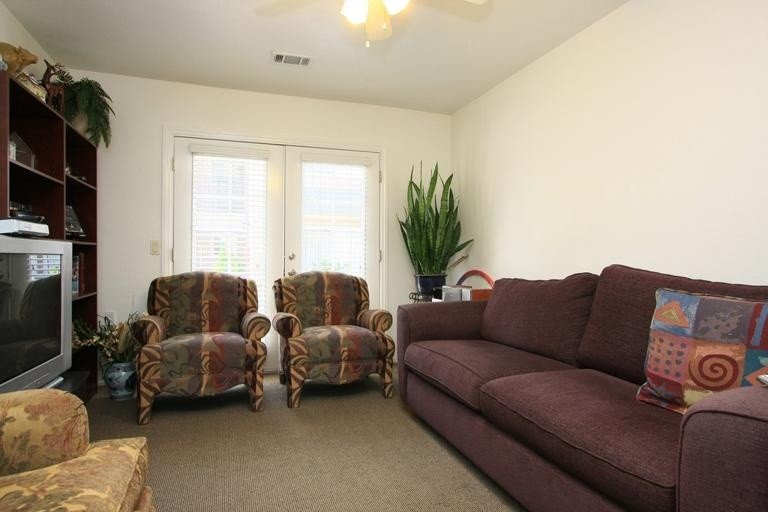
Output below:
[0,71,97,401]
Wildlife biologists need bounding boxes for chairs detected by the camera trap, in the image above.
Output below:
[0,388,155,512]
[129,271,271,425]
[271,271,395,408]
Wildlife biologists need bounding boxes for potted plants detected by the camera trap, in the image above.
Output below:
[71,309,140,400]
[395,159,475,299]
[53,71,116,149]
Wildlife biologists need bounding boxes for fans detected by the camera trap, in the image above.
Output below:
[267,0,491,43]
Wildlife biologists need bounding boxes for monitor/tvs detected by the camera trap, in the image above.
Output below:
[0,235,73,396]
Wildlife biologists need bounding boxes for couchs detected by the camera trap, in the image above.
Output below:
[397,264,768,512]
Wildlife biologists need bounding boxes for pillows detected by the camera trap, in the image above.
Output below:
[636,287,766,414]
